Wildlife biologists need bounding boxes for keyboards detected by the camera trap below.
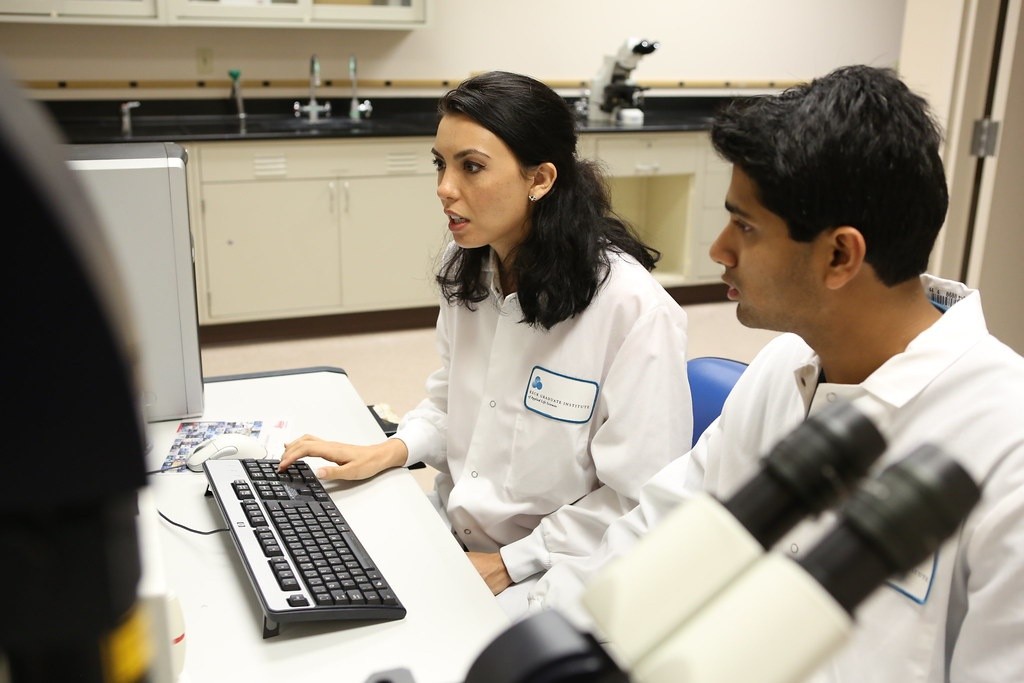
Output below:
[203,457,406,640]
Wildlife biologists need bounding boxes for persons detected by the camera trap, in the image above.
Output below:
[505,63,1024,683]
[274,70,697,615]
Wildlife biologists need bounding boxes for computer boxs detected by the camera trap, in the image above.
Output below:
[60,140,204,423]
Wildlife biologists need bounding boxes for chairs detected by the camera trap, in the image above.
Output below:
[685,357,748,450]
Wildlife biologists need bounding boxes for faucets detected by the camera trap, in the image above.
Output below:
[117,101,142,133]
[345,52,374,123]
[293,53,333,122]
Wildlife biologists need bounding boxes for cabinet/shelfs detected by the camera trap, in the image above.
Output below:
[578,133,736,289]
[0,0,429,31]
[181,142,464,326]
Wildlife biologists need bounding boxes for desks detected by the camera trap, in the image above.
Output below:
[133,365,506,682]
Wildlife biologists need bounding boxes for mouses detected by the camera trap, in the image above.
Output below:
[185,431,268,472]
